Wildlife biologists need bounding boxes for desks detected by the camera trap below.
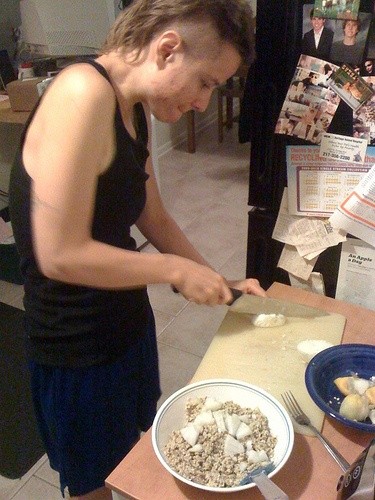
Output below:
[103,282,375,500]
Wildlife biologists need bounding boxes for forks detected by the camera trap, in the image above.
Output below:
[279,390,352,472]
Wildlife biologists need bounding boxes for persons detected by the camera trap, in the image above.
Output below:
[9,0,266,499]
[285,7,375,146]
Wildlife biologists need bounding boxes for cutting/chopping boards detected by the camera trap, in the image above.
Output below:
[189,308,347,437]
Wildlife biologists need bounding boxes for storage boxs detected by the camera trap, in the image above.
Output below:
[6,77,49,111]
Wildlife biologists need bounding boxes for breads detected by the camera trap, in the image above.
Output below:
[296,339,334,356]
[334,376,375,425]
[249,313,285,327]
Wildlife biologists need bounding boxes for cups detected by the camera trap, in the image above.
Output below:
[17,65,35,80]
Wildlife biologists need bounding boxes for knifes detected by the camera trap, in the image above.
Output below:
[170,283,330,319]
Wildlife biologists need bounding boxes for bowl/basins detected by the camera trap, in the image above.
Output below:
[305,343,375,433]
[151,379,295,492]
